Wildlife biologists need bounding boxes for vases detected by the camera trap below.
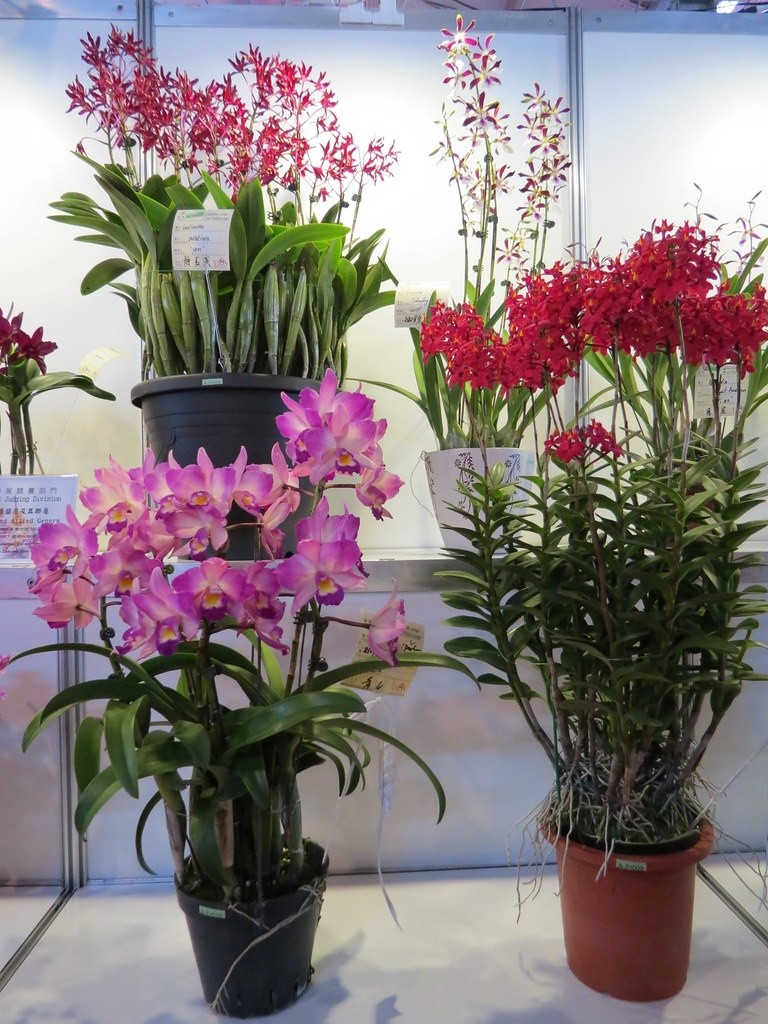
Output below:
[172,838,329,1019]
[536,806,715,1003]
[419,444,530,561]
[128,372,326,563]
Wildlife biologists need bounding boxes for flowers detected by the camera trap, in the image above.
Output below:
[414,182,768,839]
[0,300,118,476]
[345,12,571,447]
[44,22,399,387]
[0,369,482,899]
